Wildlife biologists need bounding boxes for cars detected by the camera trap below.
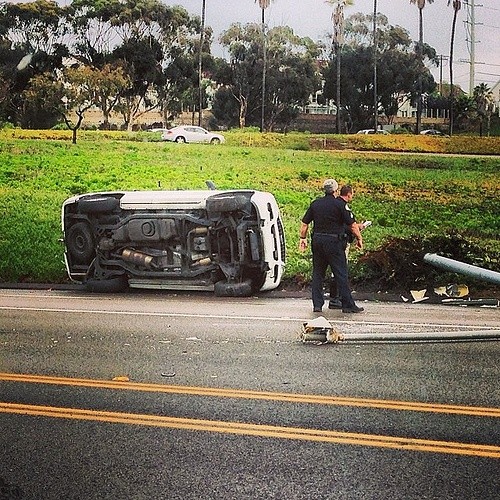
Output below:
[57,180,286,298]
[148,128,169,133]
[161,125,225,145]
[420,129,450,136]
[355,129,390,135]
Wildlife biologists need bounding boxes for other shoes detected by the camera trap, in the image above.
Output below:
[342,305,364,313]
[314,306,322,312]
[329,304,343,309]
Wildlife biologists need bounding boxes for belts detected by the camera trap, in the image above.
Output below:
[314,232,338,238]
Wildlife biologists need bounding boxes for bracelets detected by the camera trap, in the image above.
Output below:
[300,237,306,239]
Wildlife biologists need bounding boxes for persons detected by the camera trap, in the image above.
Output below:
[328,185,363,309]
[300,179,364,314]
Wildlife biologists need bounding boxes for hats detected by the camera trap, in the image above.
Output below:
[323,179,339,193]
